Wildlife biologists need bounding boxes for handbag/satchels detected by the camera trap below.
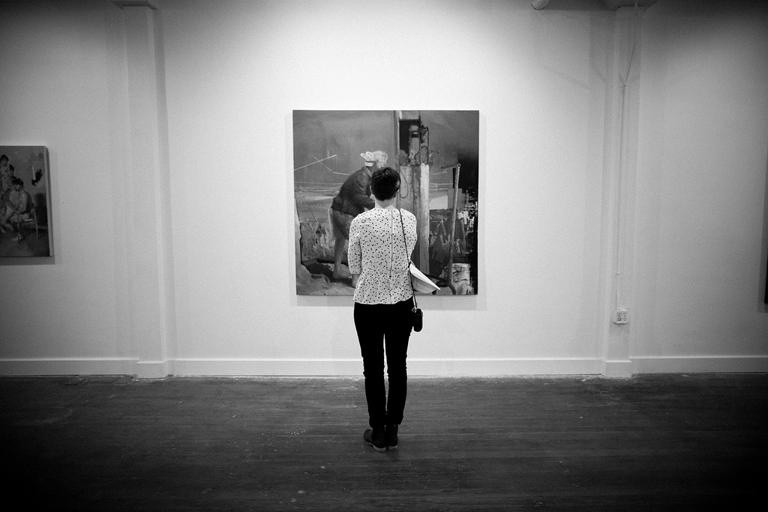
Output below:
[411,307,424,333]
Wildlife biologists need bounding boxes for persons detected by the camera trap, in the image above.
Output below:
[330,150,388,288]
[0,154,32,241]
[347,169,417,453]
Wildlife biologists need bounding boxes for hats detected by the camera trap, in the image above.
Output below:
[409,263,440,296]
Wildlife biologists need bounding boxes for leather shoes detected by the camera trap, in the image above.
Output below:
[364,424,399,452]
